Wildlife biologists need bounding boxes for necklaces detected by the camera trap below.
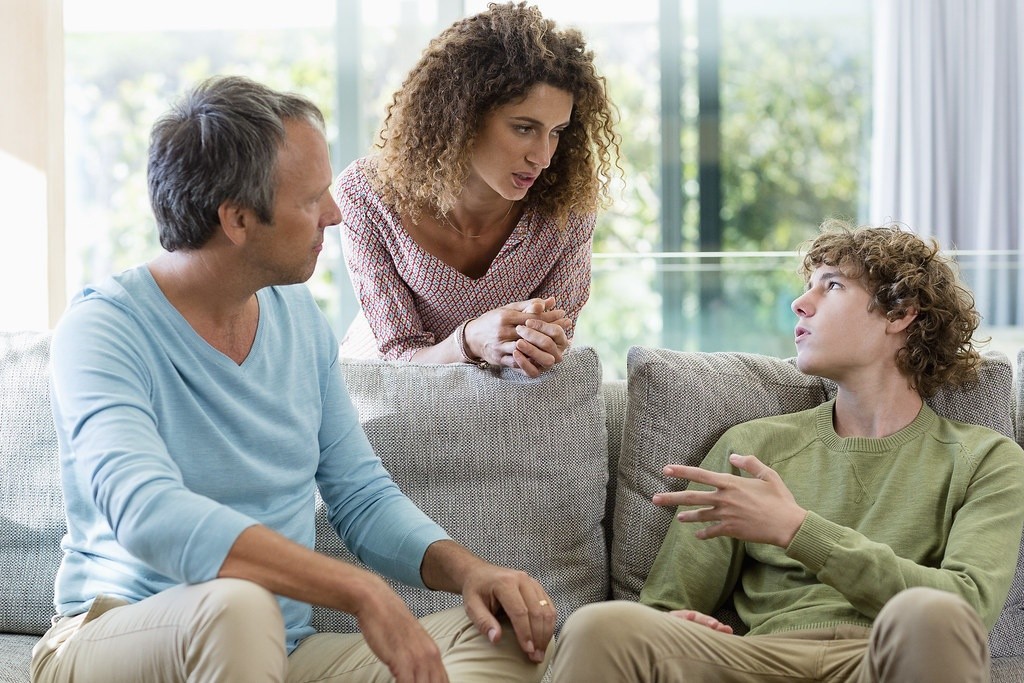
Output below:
[435,201,515,238]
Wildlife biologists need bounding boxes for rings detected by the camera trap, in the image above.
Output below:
[539,600,549,608]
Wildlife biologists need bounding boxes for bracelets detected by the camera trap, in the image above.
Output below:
[455,316,490,370]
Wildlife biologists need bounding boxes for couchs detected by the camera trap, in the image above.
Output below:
[1,348,1024,683]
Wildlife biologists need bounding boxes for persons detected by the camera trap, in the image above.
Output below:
[332,0,626,380]
[29,75,559,683]
[540,218,1024,683]
